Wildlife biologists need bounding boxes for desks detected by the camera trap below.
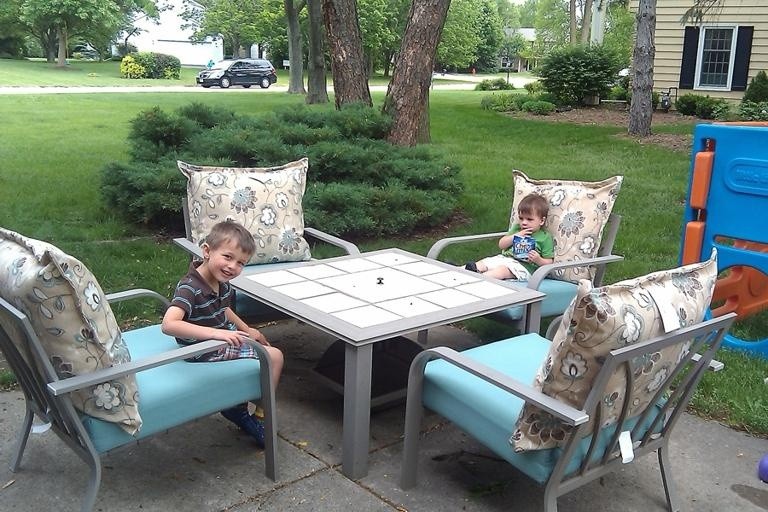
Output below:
[222,248,547,482]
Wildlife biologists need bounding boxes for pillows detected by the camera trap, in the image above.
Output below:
[1,228,144,438]
[510,168,625,288]
[176,157,316,265]
[509,244,720,453]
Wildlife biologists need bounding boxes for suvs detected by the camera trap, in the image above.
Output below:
[195,57,278,90]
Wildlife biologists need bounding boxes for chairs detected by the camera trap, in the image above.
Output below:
[172,197,361,329]
[1,287,281,507]
[415,209,627,344]
[397,311,740,511]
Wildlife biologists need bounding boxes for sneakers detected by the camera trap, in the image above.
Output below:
[221,408,266,450]
[466,262,483,273]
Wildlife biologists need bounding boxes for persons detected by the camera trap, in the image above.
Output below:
[161,219,284,449]
[465,192,554,282]
